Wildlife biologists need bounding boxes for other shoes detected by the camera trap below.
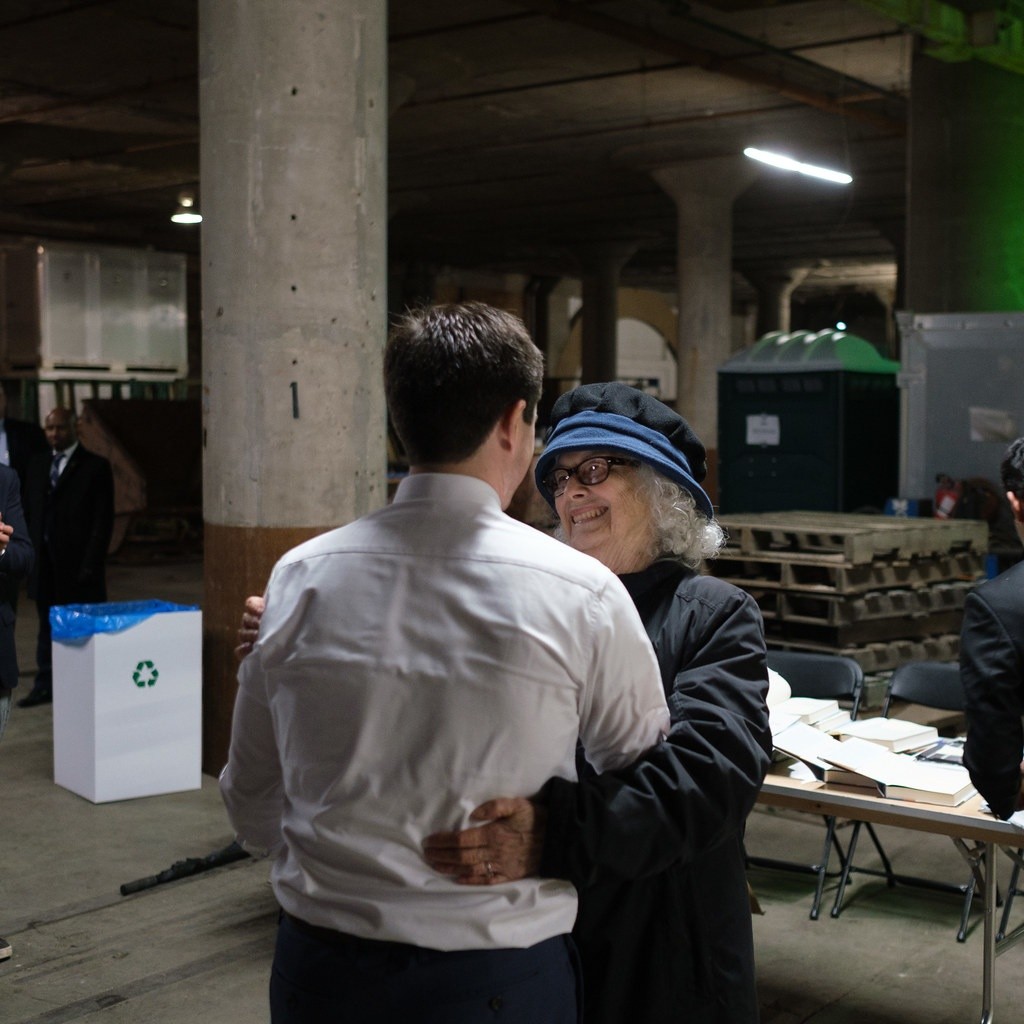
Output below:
[16,686,52,706]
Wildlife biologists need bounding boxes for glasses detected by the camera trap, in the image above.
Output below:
[542,457,641,497]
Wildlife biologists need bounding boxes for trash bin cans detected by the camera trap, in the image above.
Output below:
[50,600,203,801]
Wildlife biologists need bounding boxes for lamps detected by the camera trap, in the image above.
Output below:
[171,191,202,226]
[743,146,854,186]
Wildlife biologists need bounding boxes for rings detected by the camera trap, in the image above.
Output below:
[485,862,493,875]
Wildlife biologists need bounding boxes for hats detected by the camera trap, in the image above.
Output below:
[534,382,713,520]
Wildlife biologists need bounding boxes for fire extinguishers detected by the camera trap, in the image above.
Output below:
[933,473,963,518]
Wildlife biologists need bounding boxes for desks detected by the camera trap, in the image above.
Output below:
[760,737,1023,1024]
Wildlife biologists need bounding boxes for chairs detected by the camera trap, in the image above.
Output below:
[753,650,865,918]
[831,660,1002,943]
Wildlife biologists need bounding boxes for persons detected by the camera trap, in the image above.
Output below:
[958,436,1024,821]
[0,379,115,747]
[220,302,671,1024]
[232,382,771,1024]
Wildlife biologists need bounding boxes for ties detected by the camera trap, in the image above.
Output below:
[48,452,67,488]
